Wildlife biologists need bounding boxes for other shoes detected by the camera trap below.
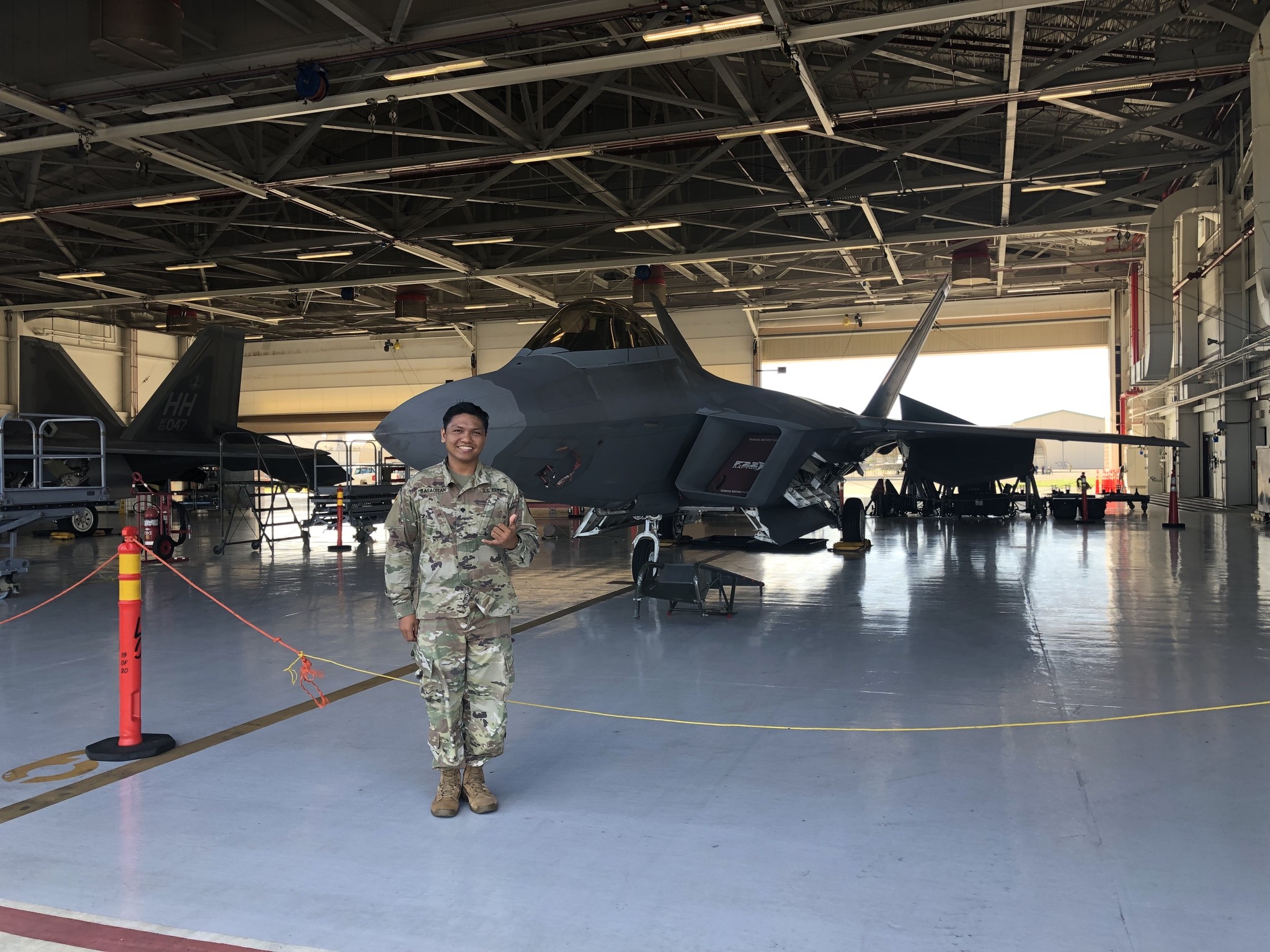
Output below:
[430,768,462,817]
[460,764,498,813]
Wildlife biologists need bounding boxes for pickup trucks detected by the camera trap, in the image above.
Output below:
[334,467,398,486]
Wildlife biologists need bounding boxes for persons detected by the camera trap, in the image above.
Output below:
[383,401,540,817]
[1048,464,1053,475]
[1035,465,1038,475]
[1068,463,1072,472]
[1041,465,1045,475]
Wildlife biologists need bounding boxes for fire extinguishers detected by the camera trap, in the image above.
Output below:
[144,503,158,550]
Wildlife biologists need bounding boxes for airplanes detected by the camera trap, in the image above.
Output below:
[373,274,1195,586]
[0,321,356,539]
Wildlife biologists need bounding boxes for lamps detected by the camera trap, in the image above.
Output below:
[1038,80,1152,101]
[57,272,106,279]
[132,196,200,207]
[452,236,513,246]
[777,204,851,216]
[141,95,234,115]
[714,121,812,141]
[0,214,36,223]
[642,12,764,42]
[614,221,681,233]
[510,146,593,164]
[165,262,217,270]
[1021,178,1106,192]
[296,250,353,259]
[383,56,490,81]
[316,173,391,183]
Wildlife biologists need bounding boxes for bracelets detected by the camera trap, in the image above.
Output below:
[517,538,521,546]
